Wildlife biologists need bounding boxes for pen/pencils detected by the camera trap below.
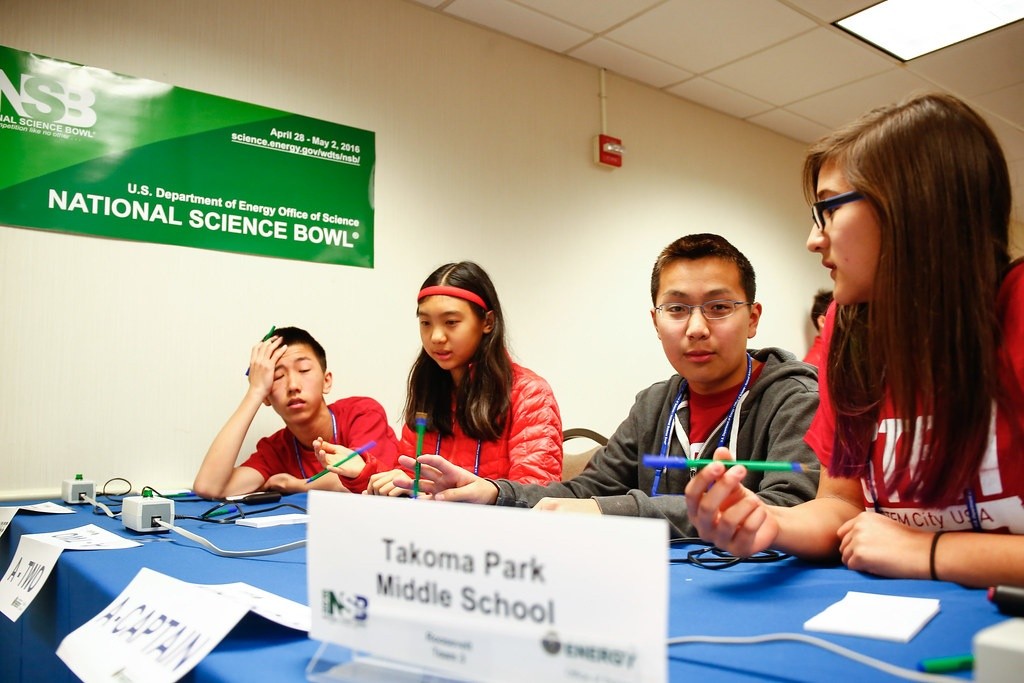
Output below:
[159,493,196,498]
[642,453,811,474]
[304,439,376,488]
[201,501,246,522]
[915,652,973,673]
[412,412,429,499]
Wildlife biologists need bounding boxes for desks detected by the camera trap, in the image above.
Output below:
[0,489,1012,683]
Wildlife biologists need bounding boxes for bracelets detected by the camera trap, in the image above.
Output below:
[928,529,953,582]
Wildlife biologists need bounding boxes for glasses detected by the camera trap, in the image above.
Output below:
[655,300,751,321]
[812,191,864,232]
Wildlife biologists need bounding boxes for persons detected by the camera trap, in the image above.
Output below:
[193,325,400,500]
[393,233,821,543]
[683,89,1023,591]
[312,262,564,498]
[804,290,836,368]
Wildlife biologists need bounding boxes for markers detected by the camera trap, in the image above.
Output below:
[987,581,1024,619]
